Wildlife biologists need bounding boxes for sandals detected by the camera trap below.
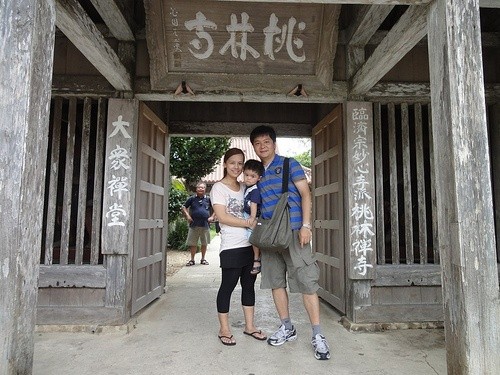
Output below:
[250,259,261,275]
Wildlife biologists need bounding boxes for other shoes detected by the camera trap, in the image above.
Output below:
[186,260,195,266]
[201,259,209,265]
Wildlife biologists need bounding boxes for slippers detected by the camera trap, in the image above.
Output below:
[218,335,236,345]
[244,330,267,340]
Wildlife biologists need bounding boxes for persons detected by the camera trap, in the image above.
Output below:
[208,125,331,360]
[182,182,216,266]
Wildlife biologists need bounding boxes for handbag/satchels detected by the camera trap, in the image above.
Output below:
[249,192,292,249]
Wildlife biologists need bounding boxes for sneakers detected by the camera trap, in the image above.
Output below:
[311,334,330,360]
[267,325,297,346]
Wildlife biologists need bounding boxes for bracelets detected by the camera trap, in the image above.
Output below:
[302,223,312,229]
